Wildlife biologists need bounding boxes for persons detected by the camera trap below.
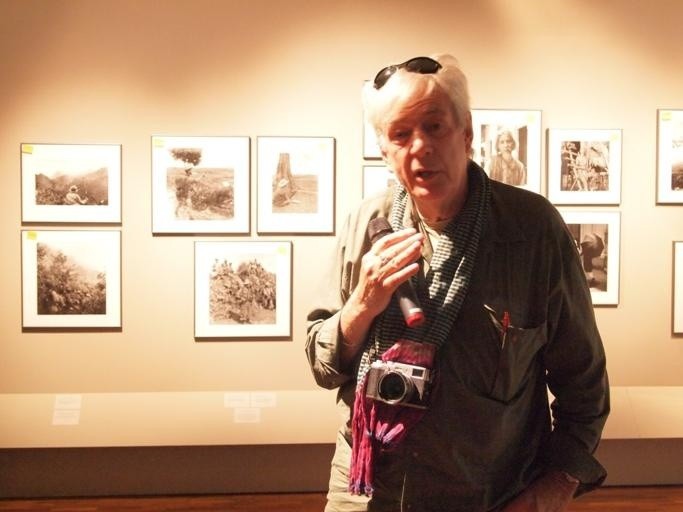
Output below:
[480,130,528,186]
[62,185,89,204]
[235,277,258,324]
[301,52,613,512]
[173,166,200,220]
[272,178,296,207]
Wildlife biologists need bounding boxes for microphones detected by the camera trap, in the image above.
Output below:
[368,218,425,327]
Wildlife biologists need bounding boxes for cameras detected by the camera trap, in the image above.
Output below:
[366,359,432,410]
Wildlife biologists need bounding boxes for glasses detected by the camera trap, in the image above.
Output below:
[373,57,442,89]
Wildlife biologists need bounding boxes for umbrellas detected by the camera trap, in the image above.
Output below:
[581,233,604,258]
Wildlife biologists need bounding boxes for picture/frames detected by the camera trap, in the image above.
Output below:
[670,240,682,336]
[361,79,387,161]
[470,106,541,198]
[20,142,123,224]
[655,107,682,205]
[19,229,123,332]
[554,209,620,307]
[256,136,337,236]
[193,240,294,339]
[361,163,396,198]
[149,134,251,236]
[546,128,620,205]
